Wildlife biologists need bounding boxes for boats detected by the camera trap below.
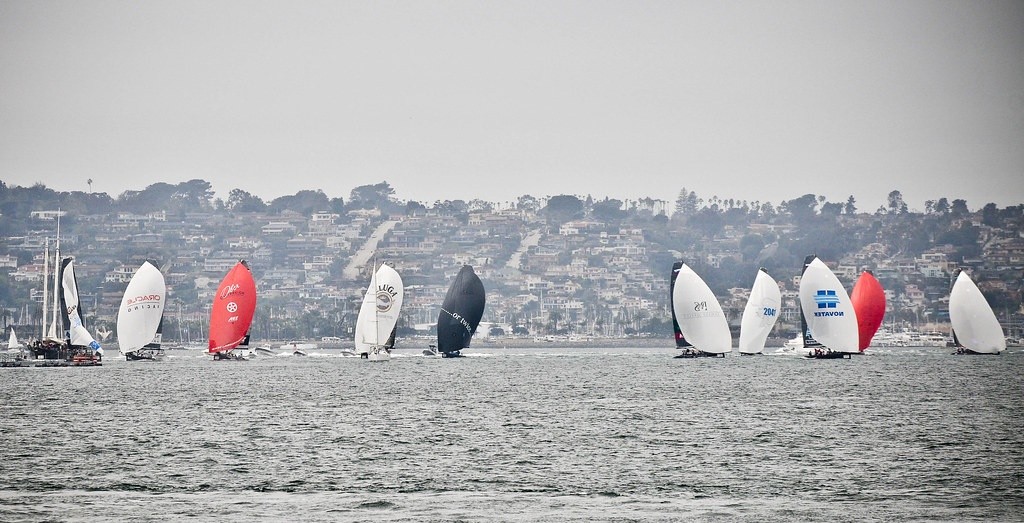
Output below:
[293,349,308,357]
[422,344,438,356]
[255,344,277,356]
[341,349,355,356]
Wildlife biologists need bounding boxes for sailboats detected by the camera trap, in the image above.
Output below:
[119,259,167,361]
[0,207,104,367]
[367,258,390,361]
[208,259,257,361]
[799,254,861,360]
[670,260,732,358]
[355,263,404,355]
[948,268,1008,356]
[738,267,781,356]
[851,271,886,355]
[437,265,485,359]
